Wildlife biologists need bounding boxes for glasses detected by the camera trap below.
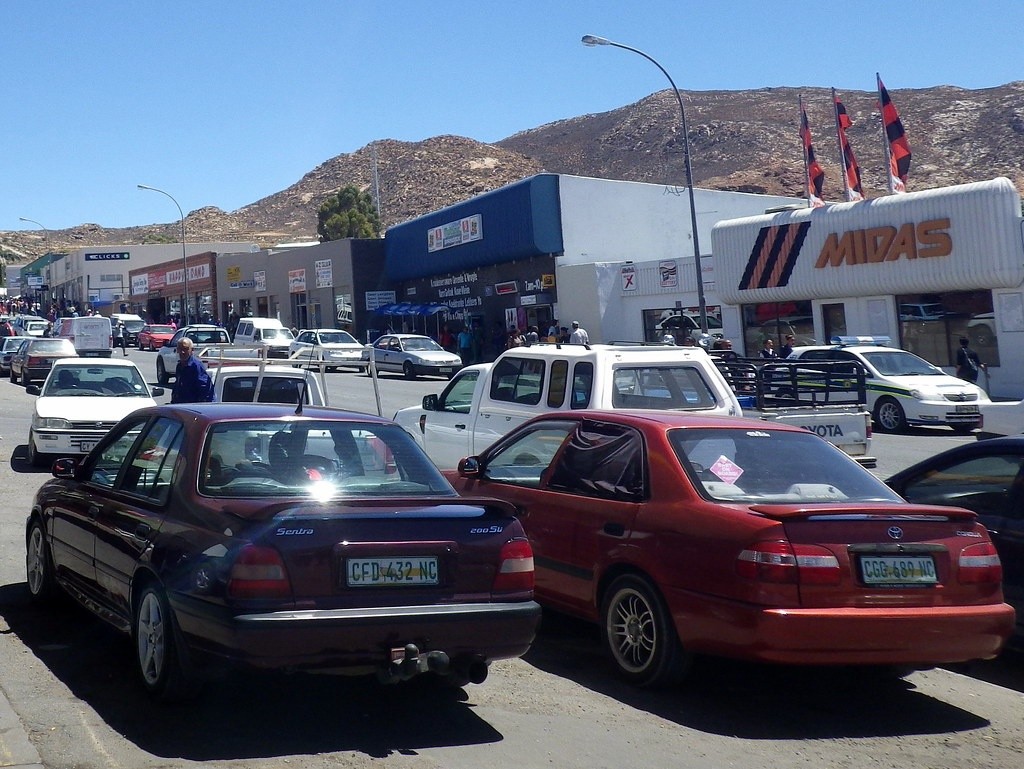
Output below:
[177,346,189,351]
[786,337,792,340]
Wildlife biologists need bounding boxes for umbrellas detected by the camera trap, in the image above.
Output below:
[375,301,451,339]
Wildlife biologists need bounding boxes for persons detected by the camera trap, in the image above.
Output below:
[167,318,177,330]
[780,334,794,358]
[113,321,130,356]
[209,315,224,326]
[956,336,984,384]
[440,320,484,365]
[709,338,750,392]
[761,339,777,378]
[163,337,216,449]
[47,303,102,322]
[43,323,55,338]
[0,296,40,316]
[492,319,589,375]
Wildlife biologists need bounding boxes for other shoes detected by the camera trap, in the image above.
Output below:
[124,353,129,356]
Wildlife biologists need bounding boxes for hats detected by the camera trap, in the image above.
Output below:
[87,309,91,313]
[571,321,578,328]
[96,311,99,313]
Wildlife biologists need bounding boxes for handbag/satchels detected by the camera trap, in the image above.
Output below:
[957,346,977,378]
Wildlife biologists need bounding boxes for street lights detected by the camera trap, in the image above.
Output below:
[138,185,189,326]
[581,35,708,334]
[20,216,53,319]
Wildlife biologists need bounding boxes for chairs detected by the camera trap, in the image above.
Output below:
[548,377,625,409]
[58,376,130,390]
[701,467,782,488]
[870,357,885,371]
[205,453,337,487]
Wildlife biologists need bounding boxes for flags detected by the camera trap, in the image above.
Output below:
[835,96,865,200]
[799,103,825,206]
[878,80,911,187]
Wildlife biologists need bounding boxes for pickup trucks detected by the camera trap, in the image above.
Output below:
[393,340,744,470]
[156,324,264,385]
[577,349,877,470]
[206,365,400,481]
[233,317,295,359]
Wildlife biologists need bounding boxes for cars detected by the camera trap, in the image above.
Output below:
[969,398,1024,441]
[654,314,723,341]
[288,329,365,372]
[361,334,464,380]
[880,434,1024,665]
[745,316,843,348]
[899,303,997,347]
[770,345,993,432]
[440,409,1017,691]
[0,315,112,385]
[25,358,164,466]
[24,403,542,703]
[110,314,176,351]
